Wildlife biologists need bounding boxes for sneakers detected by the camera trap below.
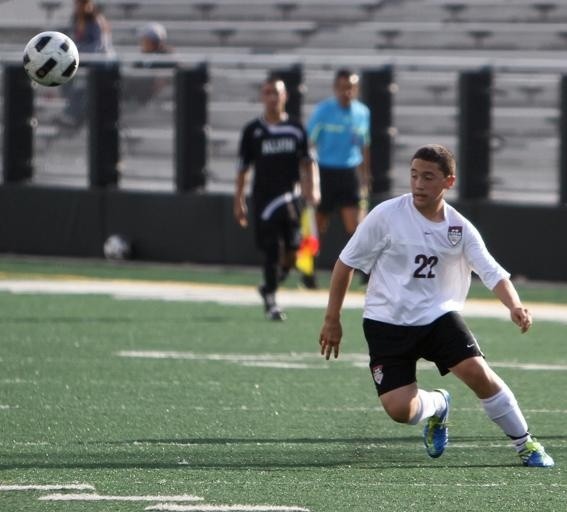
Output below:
[520,438,555,467]
[264,300,285,321]
[423,388,450,458]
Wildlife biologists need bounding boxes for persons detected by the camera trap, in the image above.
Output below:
[231,75,322,324]
[294,64,379,292]
[57,0,117,100]
[32,21,177,146]
[317,143,555,470]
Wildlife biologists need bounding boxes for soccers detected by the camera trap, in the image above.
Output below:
[103,235,131,260]
[23,31,79,86]
